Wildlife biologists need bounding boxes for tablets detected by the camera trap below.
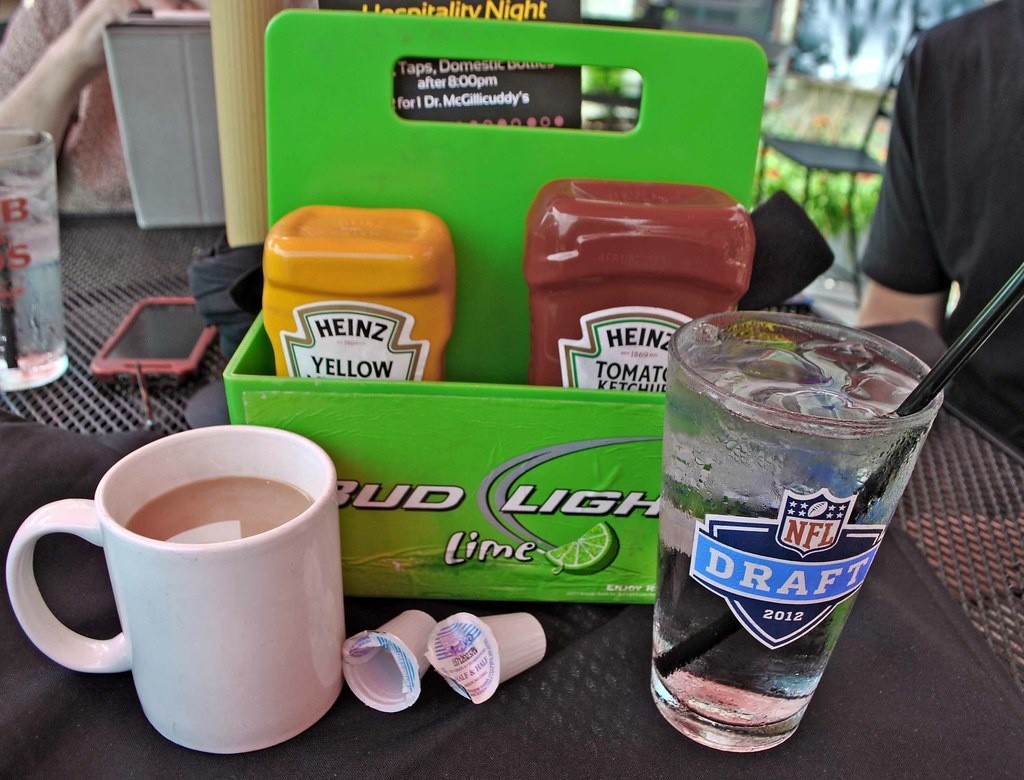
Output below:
[127,7,210,26]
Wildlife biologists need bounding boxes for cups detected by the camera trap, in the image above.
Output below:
[648,309,945,751]
[5,423,347,757]
[0,126,69,395]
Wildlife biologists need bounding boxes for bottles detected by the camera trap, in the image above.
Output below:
[263,206,458,381]
[523,177,755,391]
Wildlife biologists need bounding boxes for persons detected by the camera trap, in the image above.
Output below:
[851,0,1022,462]
[0,1,212,220]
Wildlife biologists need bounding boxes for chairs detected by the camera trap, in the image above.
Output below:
[760,23,927,310]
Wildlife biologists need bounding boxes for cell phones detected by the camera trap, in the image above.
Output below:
[91,297,218,384]
